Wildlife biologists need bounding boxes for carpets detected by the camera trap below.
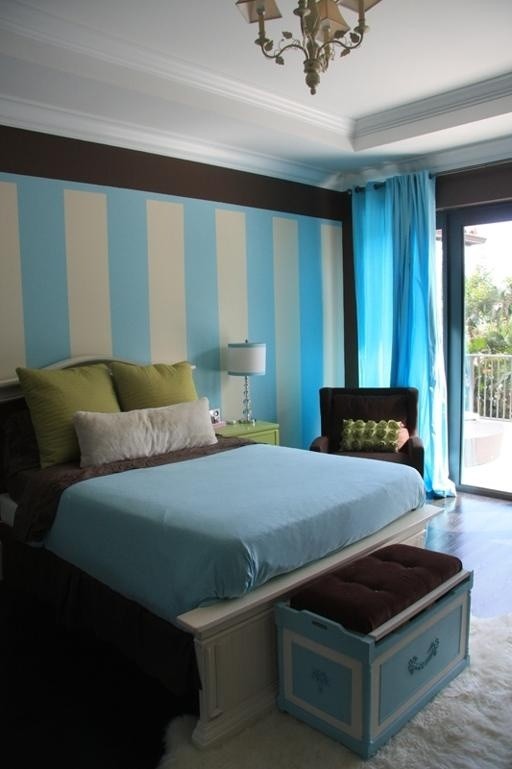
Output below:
[156,611,508,768]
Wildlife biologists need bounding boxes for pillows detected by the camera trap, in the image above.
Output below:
[9,358,217,471]
[339,417,409,453]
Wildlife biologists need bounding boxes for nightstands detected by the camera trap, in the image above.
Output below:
[214,420,279,446]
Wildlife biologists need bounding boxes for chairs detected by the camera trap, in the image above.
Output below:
[309,385,424,484]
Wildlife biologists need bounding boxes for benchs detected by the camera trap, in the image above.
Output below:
[270,540,474,765]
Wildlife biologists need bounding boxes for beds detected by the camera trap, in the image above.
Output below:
[0,355,446,723]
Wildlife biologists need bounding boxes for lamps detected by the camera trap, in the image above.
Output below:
[223,338,269,425]
[232,0,390,99]
